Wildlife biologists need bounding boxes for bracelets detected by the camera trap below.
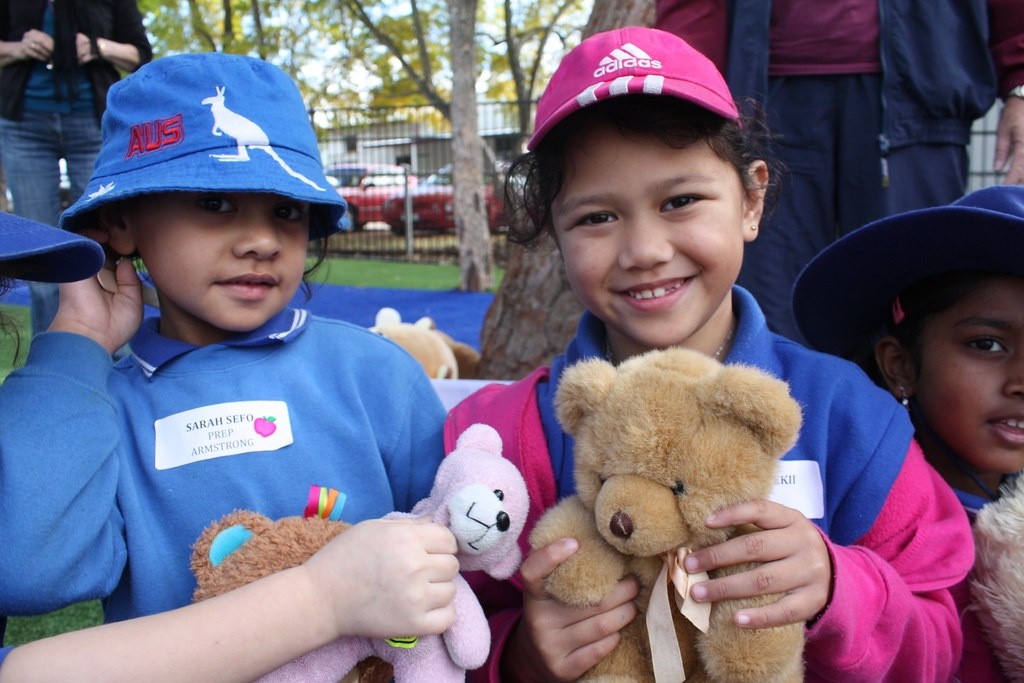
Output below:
[1008,84,1024,98]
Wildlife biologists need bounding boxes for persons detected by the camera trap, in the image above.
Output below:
[442,27,973,682]
[654,0,1024,352]
[0,213,461,683]
[1,0,153,341]
[0,53,444,629]
[790,188,1022,617]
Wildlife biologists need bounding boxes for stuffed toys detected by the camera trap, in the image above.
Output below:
[947,474,1024,683]
[367,307,481,380]
[190,506,394,681]
[527,344,809,683]
[249,423,531,683]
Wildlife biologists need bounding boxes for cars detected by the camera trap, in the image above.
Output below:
[379,159,531,236]
[325,163,423,229]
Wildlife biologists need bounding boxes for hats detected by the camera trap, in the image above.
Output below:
[527,24,741,151]
[57,51,348,240]
[0,208,106,283]
[792,184,1024,370]
